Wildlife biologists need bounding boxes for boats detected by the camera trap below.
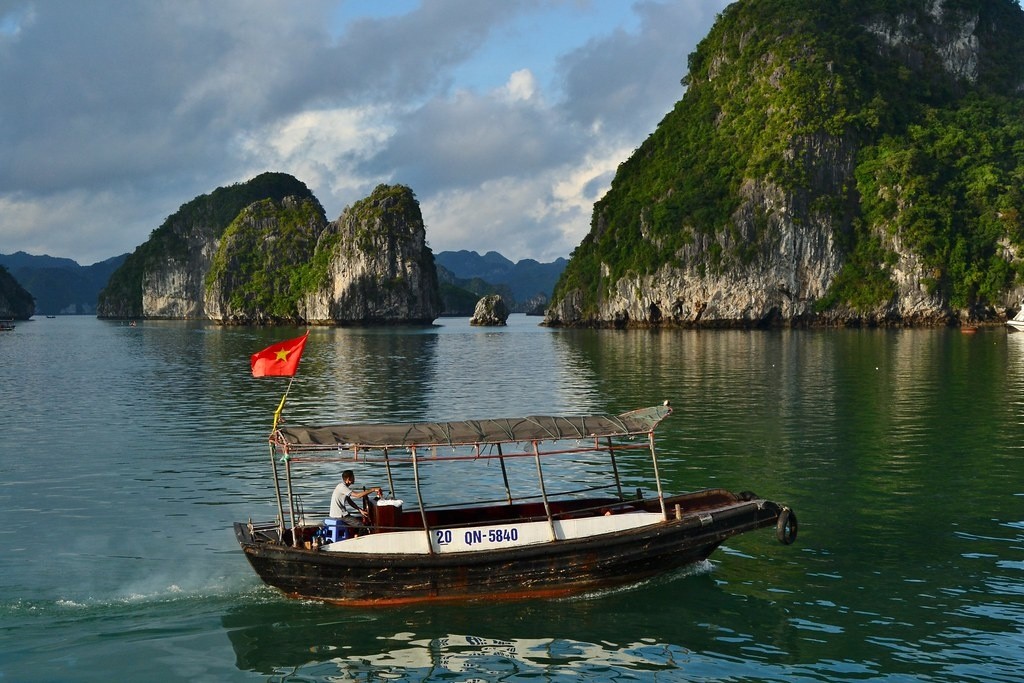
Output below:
[1004,305,1022,333]
[0,323,13,331]
[233,329,799,606]
[46,313,54,318]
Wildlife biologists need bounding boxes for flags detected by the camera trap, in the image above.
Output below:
[251,333,309,378]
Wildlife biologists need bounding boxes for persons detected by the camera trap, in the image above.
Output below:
[329,470,382,535]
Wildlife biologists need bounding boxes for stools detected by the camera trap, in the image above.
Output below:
[323,519,348,543]
